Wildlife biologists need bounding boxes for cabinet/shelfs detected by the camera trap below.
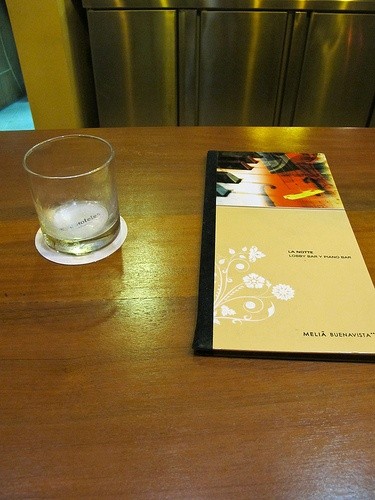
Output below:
[81,0,375,126]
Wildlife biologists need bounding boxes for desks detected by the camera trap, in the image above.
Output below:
[1,127,374,500]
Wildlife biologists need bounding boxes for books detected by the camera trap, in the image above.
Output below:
[191,151,375,363]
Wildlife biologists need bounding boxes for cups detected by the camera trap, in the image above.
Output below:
[24,136,120,255]
